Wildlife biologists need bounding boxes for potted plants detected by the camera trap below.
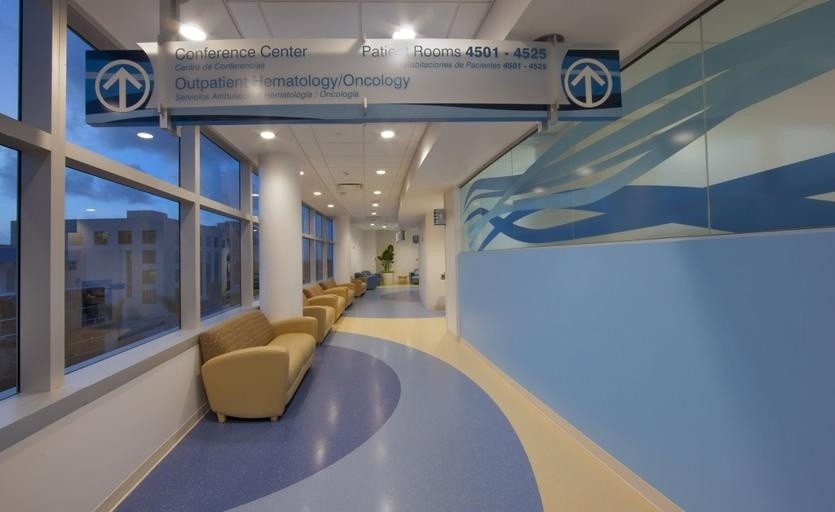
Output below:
[378,245,395,285]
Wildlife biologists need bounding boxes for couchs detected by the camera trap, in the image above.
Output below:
[410,272,419,284]
[199,309,317,421]
[303,270,380,345]
